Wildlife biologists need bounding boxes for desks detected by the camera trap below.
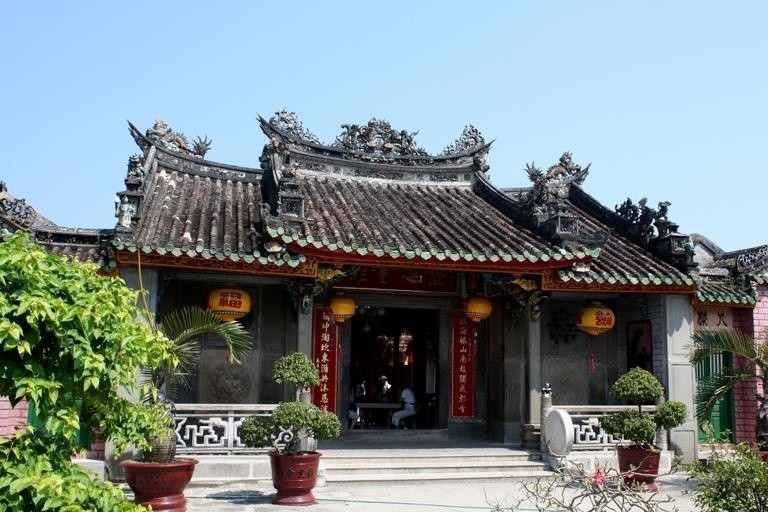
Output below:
[355,402,404,430]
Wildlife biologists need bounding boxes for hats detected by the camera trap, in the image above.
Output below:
[378,375,387,381]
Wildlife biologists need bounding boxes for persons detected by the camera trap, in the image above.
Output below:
[348,376,415,429]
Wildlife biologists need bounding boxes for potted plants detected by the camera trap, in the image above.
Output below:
[235,350,344,506]
[596,365,690,495]
[114,306,256,511]
